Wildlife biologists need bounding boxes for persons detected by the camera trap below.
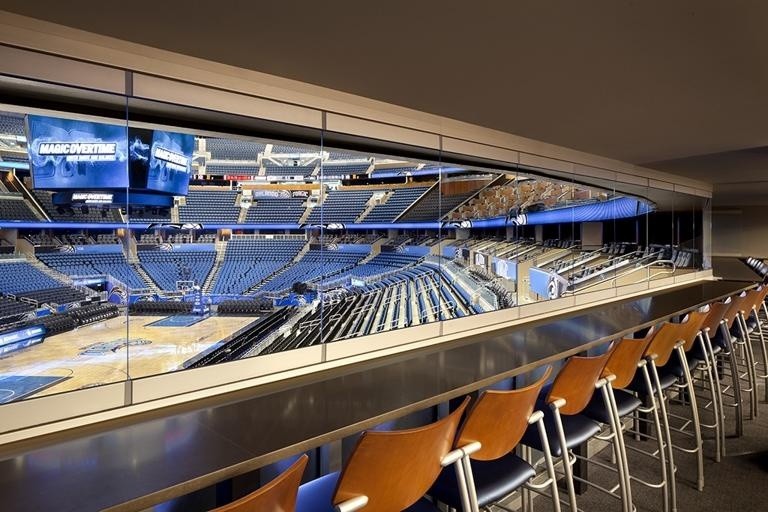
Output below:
[602,255,614,268]
[508,201,520,219]
[567,264,585,280]
[547,260,562,271]
